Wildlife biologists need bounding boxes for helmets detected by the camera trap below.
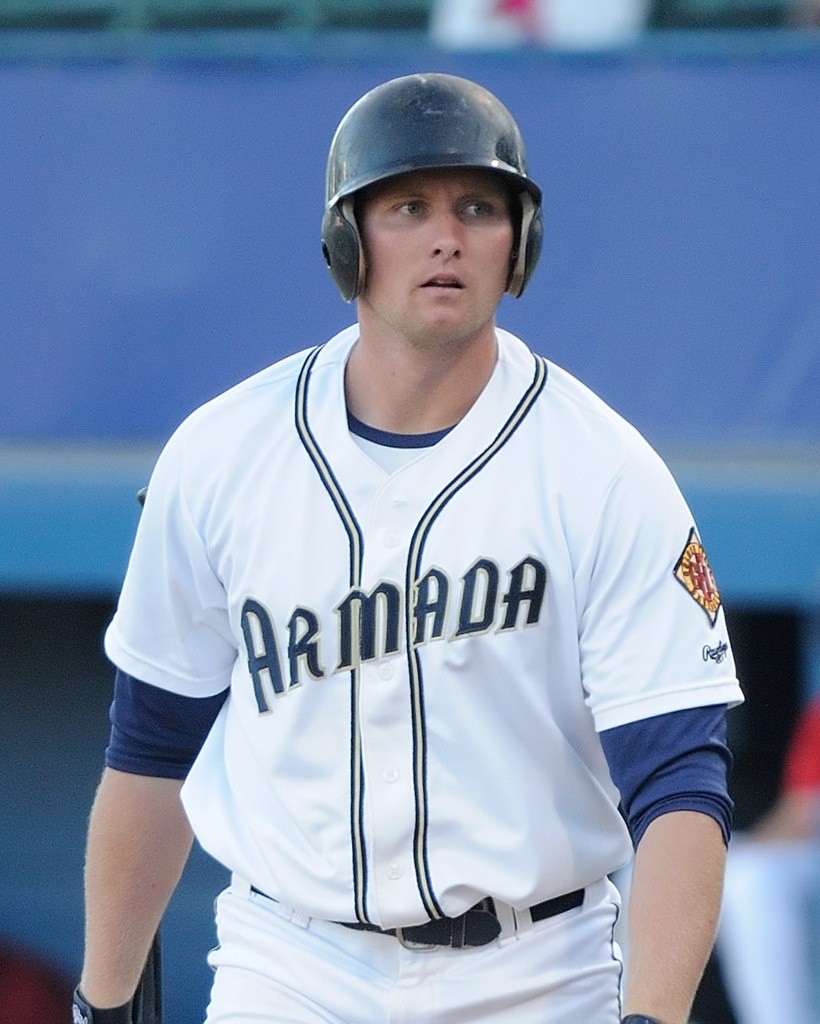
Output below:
[320,72,543,305]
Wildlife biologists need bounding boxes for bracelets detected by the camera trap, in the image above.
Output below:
[619,1014,665,1024]
[72,986,132,1024]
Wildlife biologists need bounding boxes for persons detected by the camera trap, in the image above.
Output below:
[716,696,820,1024]
[74,73,745,1024]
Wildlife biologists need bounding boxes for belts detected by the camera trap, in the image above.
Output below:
[251,884,585,951]
